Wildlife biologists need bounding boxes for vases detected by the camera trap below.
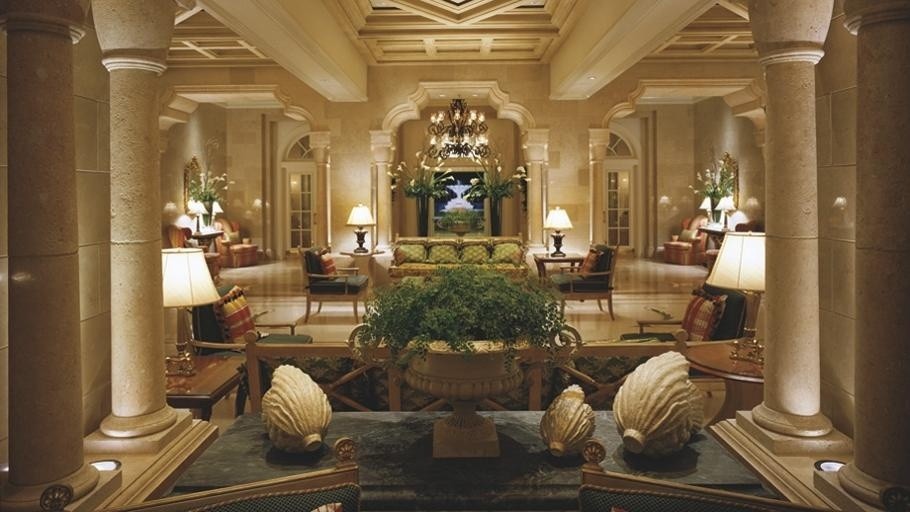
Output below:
[202,199,214,234]
[489,195,503,235]
[416,195,430,238]
[709,191,725,230]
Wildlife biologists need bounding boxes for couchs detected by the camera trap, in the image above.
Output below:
[213,216,259,270]
[662,218,709,267]
[164,223,222,279]
[387,232,530,279]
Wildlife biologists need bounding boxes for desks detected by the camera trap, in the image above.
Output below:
[533,251,585,303]
[682,340,764,428]
[162,409,779,512]
[165,355,251,424]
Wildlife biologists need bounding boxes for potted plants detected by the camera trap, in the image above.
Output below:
[437,207,487,237]
[349,252,584,460]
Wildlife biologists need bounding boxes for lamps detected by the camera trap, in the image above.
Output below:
[426,100,493,161]
[159,246,222,378]
[212,200,224,234]
[702,231,766,367]
[541,205,574,258]
[184,200,209,237]
[345,202,378,254]
[697,196,711,229]
[714,196,736,232]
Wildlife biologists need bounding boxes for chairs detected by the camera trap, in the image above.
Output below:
[619,282,749,342]
[546,240,620,322]
[296,240,370,325]
[535,328,691,412]
[109,434,364,512]
[576,437,831,512]
[191,282,315,356]
[243,329,395,411]
[393,338,542,411]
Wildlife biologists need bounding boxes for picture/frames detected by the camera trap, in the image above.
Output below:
[426,165,493,238]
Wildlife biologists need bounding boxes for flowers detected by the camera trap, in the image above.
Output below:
[386,149,455,231]
[181,169,237,226]
[686,151,738,223]
[463,155,516,232]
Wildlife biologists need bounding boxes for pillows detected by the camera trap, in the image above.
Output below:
[680,285,730,342]
[491,238,525,265]
[318,253,340,282]
[226,230,240,246]
[393,240,428,267]
[428,240,459,264]
[459,240,492,264]
[678,228,699,243]
[578,246,605,283]
[212,284,270,354]
